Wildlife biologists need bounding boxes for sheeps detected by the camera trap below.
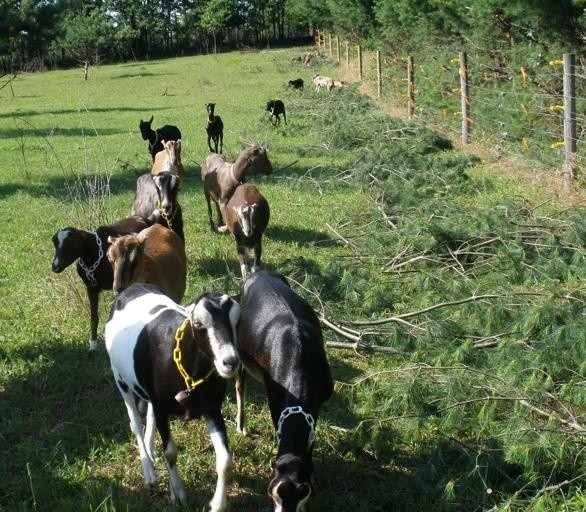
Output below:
[150,140,184,191]
[201,146,273,232]
[51,215,154,360]
[311,73,345,97]
[139,115,182,165]
[204,103,224,155]
[232,269,335,512]
[286,78,304,94]
[266,99,287,126]
[106,222,186,305]
[130,171,185,241]
[225,183,270,283]
[104,282,242,512]
